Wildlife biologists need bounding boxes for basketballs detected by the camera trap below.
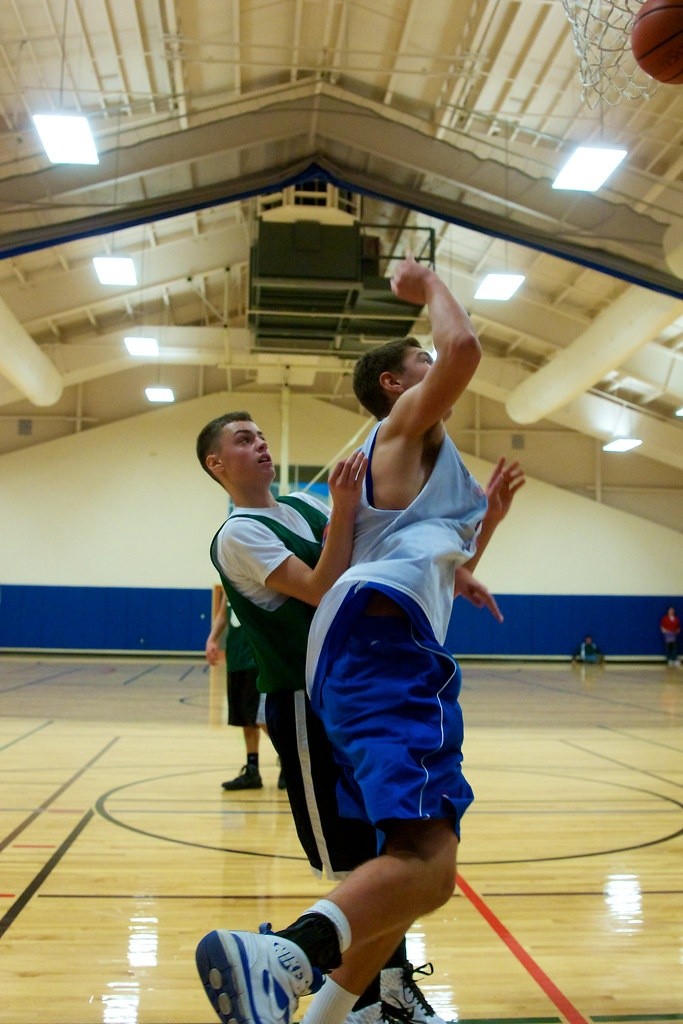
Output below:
[631,1,683,84]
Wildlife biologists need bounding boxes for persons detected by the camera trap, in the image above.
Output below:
[660,607,681,666]
[203,588,288,790]
[572,635,603,666]
[196,412,525,1024]
[194,252,503,1024]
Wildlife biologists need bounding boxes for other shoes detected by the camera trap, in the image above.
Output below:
[222,765,262,790]
[277,755,287,789]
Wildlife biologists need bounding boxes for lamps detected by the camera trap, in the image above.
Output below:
[29,0,100,166]
[92,94,138,287]
[549,0,629,194]
[601,380,643,454]
[122,225,160,359]
[142,300,176,404]
[473,119,524,301]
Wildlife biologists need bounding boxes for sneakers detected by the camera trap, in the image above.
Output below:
[380,962,448,1024]
[345,999,405,1024]
[196,922,326,1024]
